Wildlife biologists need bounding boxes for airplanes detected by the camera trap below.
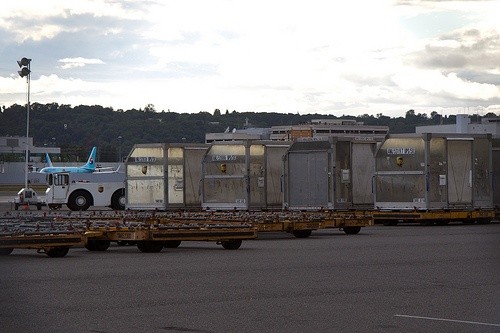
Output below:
[39,147,112,174]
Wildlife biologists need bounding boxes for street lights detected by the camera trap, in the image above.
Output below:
[14,57,42,211]
